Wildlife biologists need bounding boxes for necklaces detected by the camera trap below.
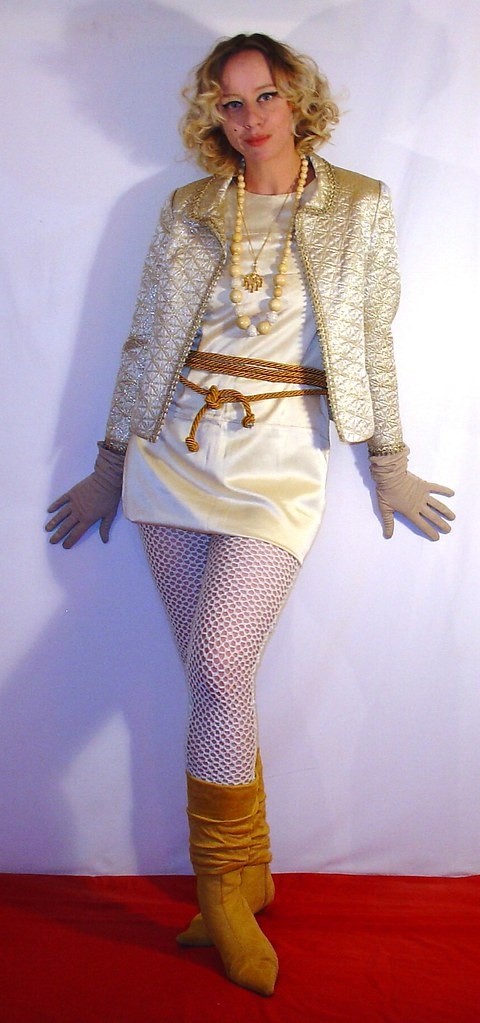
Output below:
[228,154,308,340]
[236,177,298,293]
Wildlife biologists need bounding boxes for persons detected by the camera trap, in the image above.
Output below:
[43,32,457,997]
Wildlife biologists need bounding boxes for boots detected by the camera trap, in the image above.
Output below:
[185,770,279,996]
[177,749,275,946]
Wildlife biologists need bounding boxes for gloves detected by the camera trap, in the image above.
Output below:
[367,444,455,540]
[45,441,126,549]
